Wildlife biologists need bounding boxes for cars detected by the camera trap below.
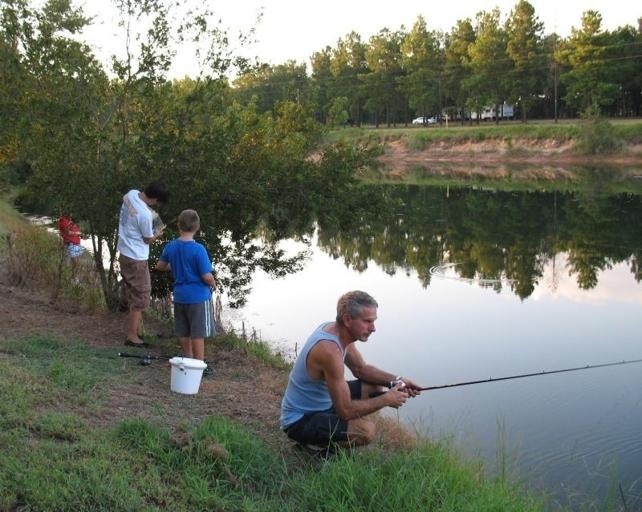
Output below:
[412,116,437,125]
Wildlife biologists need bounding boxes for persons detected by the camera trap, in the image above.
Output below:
[280,291,423,460]
[59,216,84,283]
[117,181,169,348]
[156,209,217,375]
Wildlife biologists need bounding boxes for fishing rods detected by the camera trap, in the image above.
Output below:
[370,359,642,399]
[118,353,217,366]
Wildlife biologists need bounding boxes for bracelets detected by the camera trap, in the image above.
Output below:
[389,376,404,387]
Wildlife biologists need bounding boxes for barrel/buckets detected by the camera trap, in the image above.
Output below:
[168,356,208,396]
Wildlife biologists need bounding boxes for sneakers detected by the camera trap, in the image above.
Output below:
[203,367,213,376]
[292,443,335,462]
[124,335,151,348]
